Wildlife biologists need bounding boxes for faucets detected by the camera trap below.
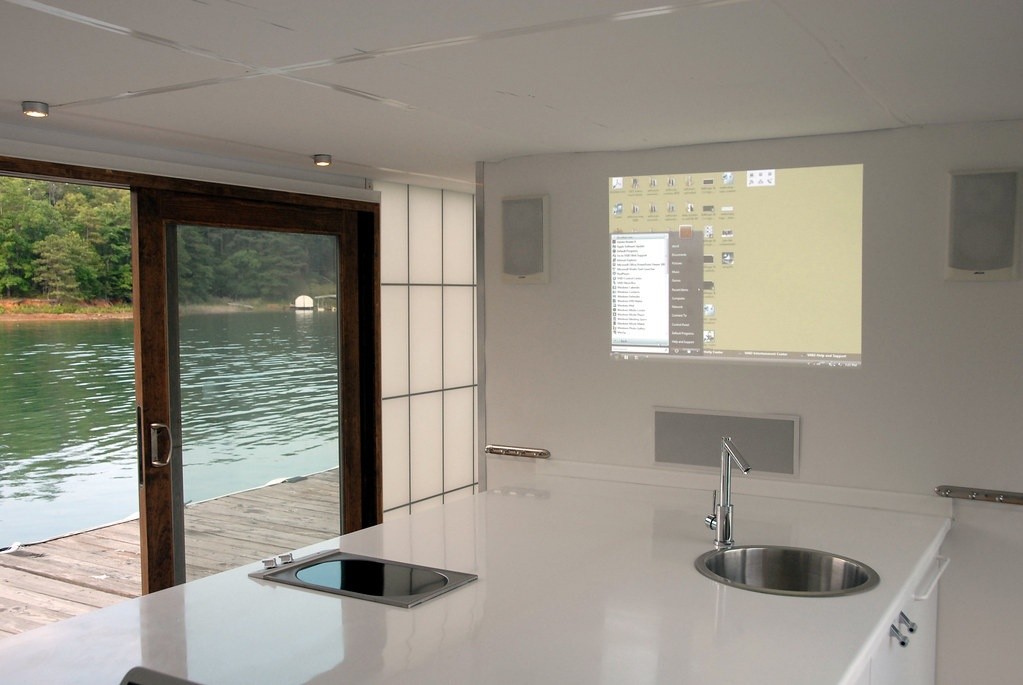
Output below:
[705,436,753,549]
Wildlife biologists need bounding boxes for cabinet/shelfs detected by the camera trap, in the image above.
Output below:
[856,545,950,685]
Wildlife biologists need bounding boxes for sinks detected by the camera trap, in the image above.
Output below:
[695,546,882,597]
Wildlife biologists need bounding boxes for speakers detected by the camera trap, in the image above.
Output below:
[501,193,550,285]
[945,169,1020,280]
